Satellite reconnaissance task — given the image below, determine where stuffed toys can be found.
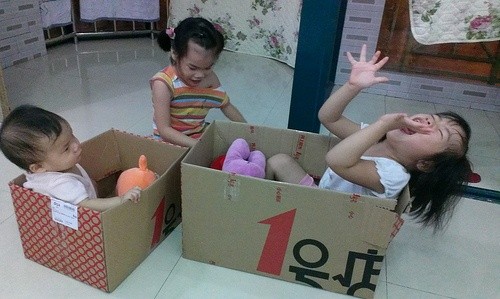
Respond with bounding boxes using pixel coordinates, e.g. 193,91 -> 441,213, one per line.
221,138 -> 266,179
116,155 -> 158,197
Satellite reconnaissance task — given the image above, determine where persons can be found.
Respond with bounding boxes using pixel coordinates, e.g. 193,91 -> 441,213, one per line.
266,43 -> 471,235
0,105 -> 143,212
148,17 -> 248,148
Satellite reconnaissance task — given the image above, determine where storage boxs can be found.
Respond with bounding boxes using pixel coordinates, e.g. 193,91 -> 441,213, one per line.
181,121 -> 411,299
9,128 -> 192,293
